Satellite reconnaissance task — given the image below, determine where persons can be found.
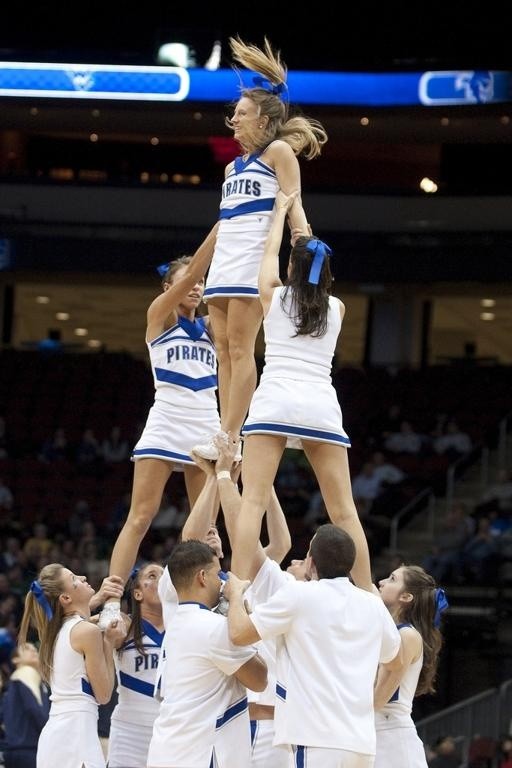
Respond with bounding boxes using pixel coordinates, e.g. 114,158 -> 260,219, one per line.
0,330 -> 512,768
216,188 -> 372,614
97,220 -> 221,637
193,33 -> 328,462
